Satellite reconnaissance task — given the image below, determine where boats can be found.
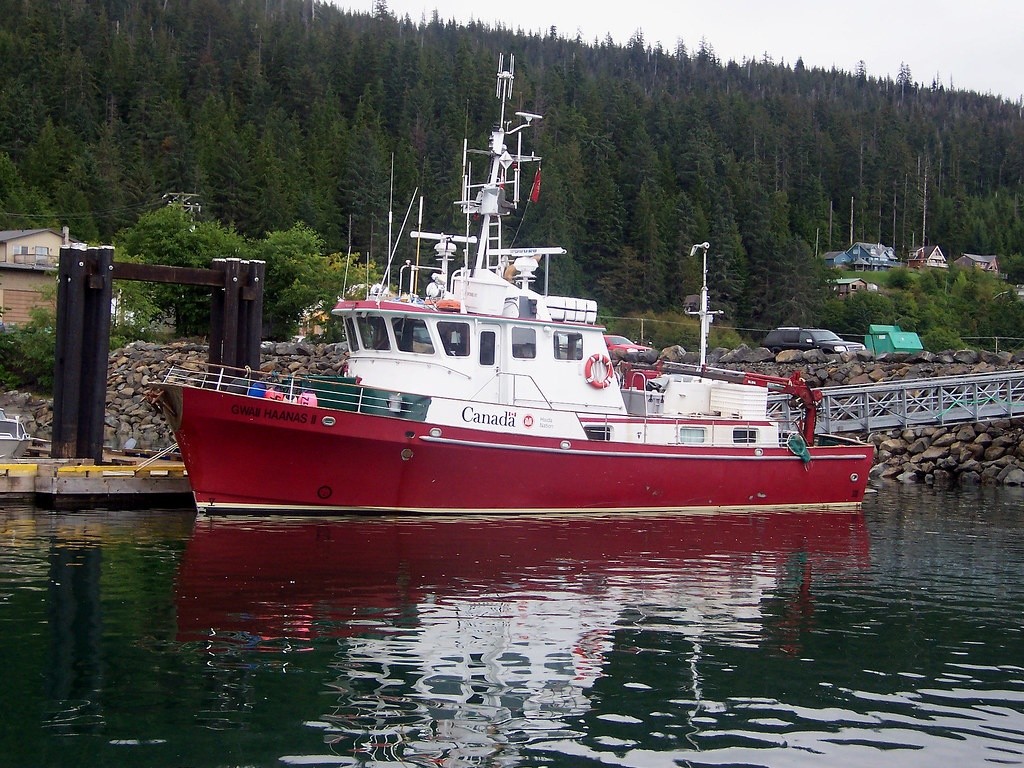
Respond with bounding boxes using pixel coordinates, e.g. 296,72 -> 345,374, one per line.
141,49 -> 876,515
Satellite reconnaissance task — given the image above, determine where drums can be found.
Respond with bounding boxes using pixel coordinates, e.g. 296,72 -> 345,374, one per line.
585,354 -> 613,388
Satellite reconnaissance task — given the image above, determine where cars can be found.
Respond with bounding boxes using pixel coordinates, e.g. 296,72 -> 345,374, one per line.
601,333 -> 653,356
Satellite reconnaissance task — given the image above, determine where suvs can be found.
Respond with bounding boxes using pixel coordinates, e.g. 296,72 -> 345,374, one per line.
759,327 -> 865,354
